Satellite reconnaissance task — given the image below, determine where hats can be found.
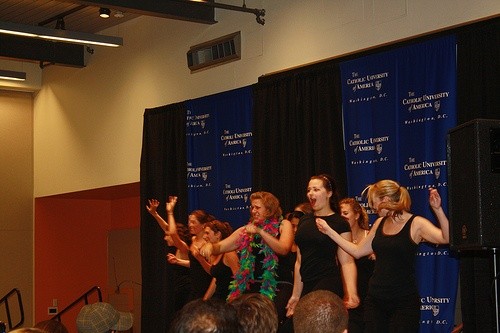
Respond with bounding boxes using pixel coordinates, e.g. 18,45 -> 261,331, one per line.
76,302 -> 134,333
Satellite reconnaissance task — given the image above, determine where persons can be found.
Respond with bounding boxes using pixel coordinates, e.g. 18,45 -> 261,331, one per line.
76,301 -> 135,333
204,221 -> 241,301
274,201 -> 312,333
314,187 -> 449,333
340,197 -> 376,333
229,292 -> 278,333
291,290 -> 348,333
167,297 -> 241,333
452,224 -> 475,245
285,174 -> 360,316
199,191 -> 293,304
166,210 -> 216,300
146,195 -> 193,324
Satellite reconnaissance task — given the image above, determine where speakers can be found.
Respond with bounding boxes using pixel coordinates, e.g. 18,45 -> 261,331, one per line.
446,118 -> 500,253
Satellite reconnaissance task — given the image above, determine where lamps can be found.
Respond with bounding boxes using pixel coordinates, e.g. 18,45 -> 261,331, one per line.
0,14 -> 123,49
99,8 -> 111,19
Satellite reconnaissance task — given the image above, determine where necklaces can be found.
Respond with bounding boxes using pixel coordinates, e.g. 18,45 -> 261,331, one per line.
351,230 -> 365,244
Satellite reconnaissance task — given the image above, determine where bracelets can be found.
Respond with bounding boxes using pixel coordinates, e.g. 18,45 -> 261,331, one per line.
167,212 -> 174,215
